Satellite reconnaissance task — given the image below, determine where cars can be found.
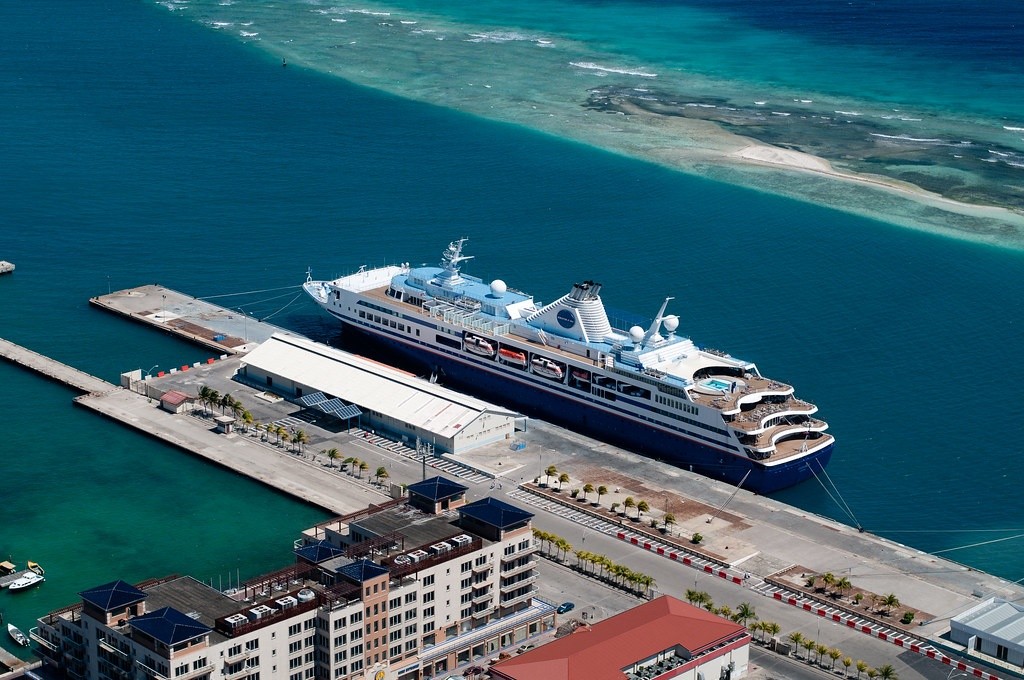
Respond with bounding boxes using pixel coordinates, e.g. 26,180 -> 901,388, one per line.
463,665 -> 484,678
517,643 -> 535,654
557,602 -> 575,614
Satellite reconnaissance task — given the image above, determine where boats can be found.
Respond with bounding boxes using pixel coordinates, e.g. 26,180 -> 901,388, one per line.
8,623 -> 31,647
8,571 -> 45,592
27,560 -> 44,576
302,233 -> 836,495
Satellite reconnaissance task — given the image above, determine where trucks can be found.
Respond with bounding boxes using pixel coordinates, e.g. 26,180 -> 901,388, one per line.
489,651 -> 512,664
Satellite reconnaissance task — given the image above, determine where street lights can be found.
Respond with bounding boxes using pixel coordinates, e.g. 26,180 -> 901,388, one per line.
303,419 -> 318,457
107,275 -> 110,297
238,307 -> 254,341
817,617 -> 820,643
219,388 -> 239,416
136,364 -> 161,403
